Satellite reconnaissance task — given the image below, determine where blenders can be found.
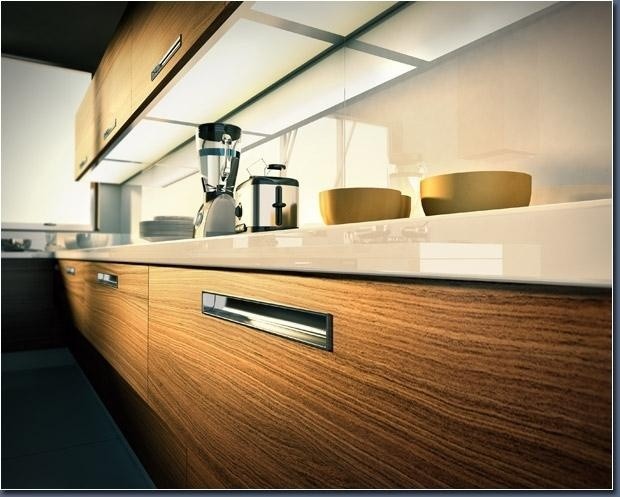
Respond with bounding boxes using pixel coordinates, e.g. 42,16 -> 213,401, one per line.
194,123 -> 242,239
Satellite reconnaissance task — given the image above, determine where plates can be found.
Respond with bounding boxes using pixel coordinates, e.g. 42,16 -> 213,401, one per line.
138,215 -> 194,242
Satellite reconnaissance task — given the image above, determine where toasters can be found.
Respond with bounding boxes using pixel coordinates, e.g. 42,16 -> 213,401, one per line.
234,164 -> 300,232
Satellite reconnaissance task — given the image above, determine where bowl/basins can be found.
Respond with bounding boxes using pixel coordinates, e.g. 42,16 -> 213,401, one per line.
319,188 -> 411,225
1,238 -> 32,251
420,171 -> 531,215
64,232 -> 111,248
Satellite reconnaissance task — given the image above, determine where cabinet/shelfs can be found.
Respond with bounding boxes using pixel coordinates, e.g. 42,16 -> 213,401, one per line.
74,0 -> 408,186
3,260 -> 63,353
55,260 -> 613,489
